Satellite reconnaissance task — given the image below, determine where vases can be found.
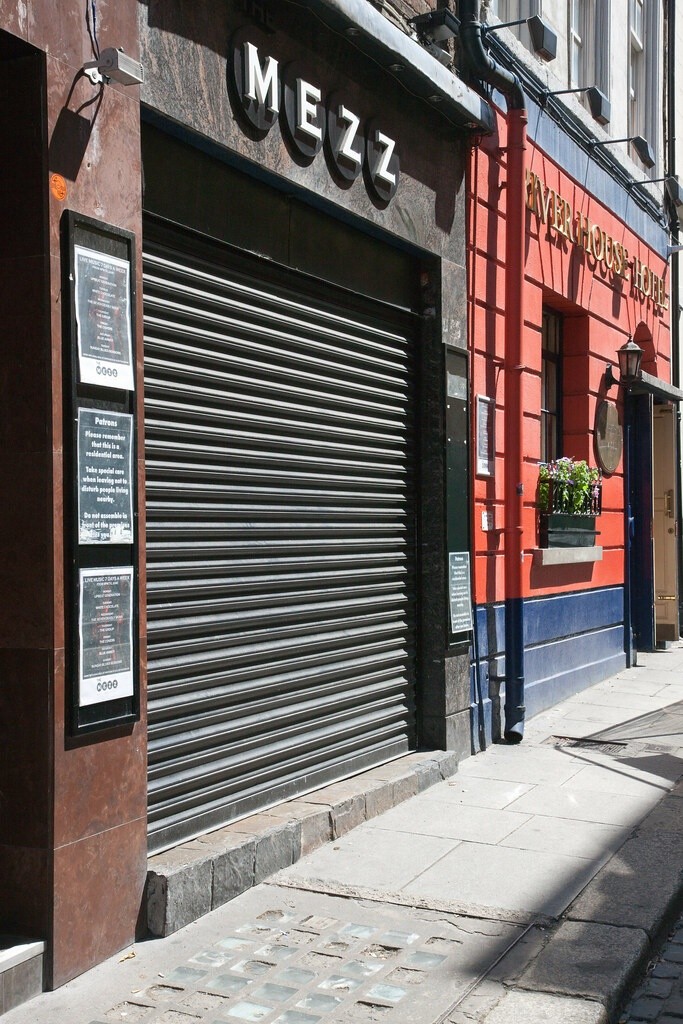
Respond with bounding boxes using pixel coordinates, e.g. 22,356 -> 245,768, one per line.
540,514 -> 596,548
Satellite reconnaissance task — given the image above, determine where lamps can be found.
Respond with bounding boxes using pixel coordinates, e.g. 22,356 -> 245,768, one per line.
629,176 -> 683,208
606,332 -> 645,390
482,14 -> 558,61
540,85 -> 611,125
589,135 -> 656,169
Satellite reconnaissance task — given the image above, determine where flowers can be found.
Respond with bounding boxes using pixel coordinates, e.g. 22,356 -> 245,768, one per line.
540,455 -> 603,517
